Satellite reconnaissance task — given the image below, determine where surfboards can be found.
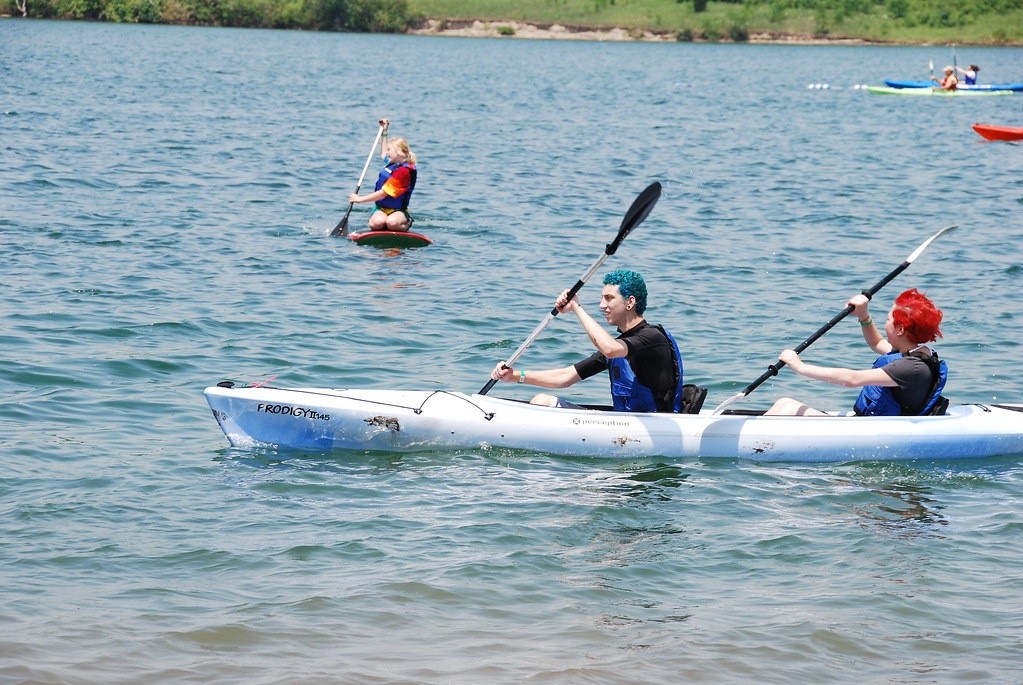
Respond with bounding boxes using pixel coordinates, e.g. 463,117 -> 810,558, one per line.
345,224 -> 433,247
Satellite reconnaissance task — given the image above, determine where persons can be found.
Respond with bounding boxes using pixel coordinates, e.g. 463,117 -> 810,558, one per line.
930,68 -> 958,92
491,270 -> 676,412
777,286 -> 944,416
349,119 -> 417,232
957,64 -> 980,85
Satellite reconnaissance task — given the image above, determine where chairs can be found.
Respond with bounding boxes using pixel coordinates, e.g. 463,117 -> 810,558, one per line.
681,383 -> 708,413
926,395 -> 950,416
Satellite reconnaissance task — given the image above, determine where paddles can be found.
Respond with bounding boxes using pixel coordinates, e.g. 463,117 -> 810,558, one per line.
929,59 -> 935,87
713,225 -> 961,416
329,126 -> 383,237
954,55 -> 957,77
478,180 -> 662,396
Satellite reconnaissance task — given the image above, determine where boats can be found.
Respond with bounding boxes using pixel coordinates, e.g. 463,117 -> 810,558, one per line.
203,384 -> 1022,464
973,124 -> 1023,142
867,81 -> 1023,96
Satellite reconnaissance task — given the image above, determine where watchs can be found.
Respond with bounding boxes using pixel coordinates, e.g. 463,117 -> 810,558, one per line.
517,369 -> 526,384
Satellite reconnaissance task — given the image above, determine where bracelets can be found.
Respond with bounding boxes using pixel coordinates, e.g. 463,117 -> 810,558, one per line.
858,314 -> 873,326
381,134 -> 387,136
